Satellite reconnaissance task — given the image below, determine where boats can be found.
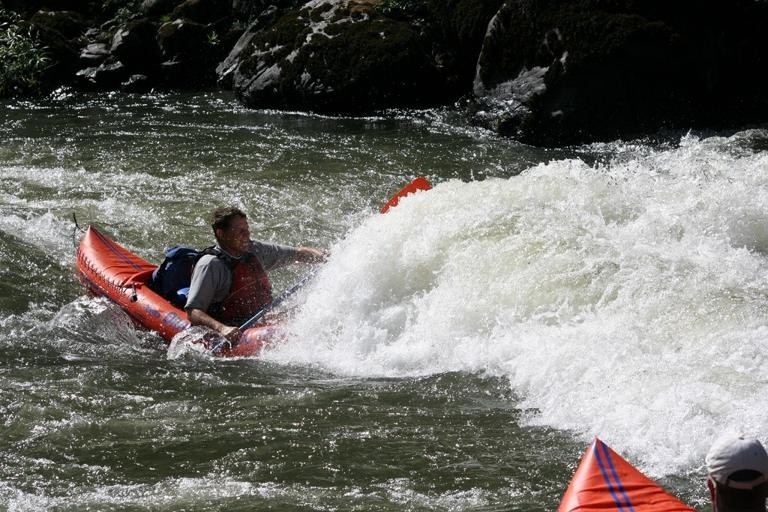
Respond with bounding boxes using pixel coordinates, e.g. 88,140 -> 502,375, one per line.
549,434 -> 696,512
73,218 -> 287,364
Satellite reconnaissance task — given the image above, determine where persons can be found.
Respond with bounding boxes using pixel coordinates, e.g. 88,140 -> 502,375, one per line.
184,203 -> 328,345
703,433 -> 768,512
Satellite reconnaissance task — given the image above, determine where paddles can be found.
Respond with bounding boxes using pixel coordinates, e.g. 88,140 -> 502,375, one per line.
210,177 -> 432,352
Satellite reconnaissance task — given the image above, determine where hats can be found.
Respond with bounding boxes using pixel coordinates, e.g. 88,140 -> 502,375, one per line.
705,433 -> 768,489
